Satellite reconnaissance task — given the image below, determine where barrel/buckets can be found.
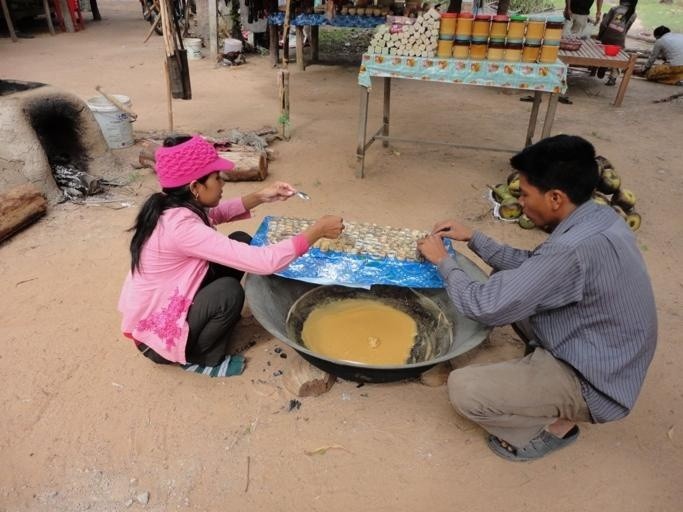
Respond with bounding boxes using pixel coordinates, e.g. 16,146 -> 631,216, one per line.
86,94 -> 135,150
223,38 -> 242,64
183,38 -> 202,61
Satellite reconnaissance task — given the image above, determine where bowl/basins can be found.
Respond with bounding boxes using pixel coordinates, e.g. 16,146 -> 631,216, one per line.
604,44 -> 620,56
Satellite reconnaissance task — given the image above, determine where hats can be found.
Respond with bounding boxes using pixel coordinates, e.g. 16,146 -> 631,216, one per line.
155,133 -> 234,189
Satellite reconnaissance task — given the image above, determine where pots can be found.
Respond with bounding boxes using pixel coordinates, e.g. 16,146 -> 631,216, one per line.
243,245 -> 499,387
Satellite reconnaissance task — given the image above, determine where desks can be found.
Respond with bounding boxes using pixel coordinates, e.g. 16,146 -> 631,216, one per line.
267,12 -> 386,72
352,53 -> 567,183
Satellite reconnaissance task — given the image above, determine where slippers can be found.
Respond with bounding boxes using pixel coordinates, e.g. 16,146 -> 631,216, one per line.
488,424 -> 580,462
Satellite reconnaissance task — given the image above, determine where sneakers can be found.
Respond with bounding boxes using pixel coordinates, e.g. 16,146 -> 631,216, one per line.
520,96 -> 541,101
597,67 -> 609,78
559,96 -> 573,105
608,78 -> 615,85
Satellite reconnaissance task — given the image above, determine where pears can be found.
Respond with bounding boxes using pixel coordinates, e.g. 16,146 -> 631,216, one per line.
486,156 -> 641,232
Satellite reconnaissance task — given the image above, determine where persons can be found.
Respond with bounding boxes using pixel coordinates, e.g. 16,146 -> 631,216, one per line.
596,0 -> 638,86
563,0 -> 603,34
415,134 -> 659,463
633,26 -> 683,87
116,133 -> 347,378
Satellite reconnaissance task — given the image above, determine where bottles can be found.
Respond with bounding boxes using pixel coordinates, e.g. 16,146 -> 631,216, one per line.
438,13 -> 564,65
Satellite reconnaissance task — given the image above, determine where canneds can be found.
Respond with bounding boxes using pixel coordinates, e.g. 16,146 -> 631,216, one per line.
438,11 -> 564,64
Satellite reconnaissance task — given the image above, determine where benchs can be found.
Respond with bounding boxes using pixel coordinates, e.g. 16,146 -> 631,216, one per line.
555,35 -> 638,108
